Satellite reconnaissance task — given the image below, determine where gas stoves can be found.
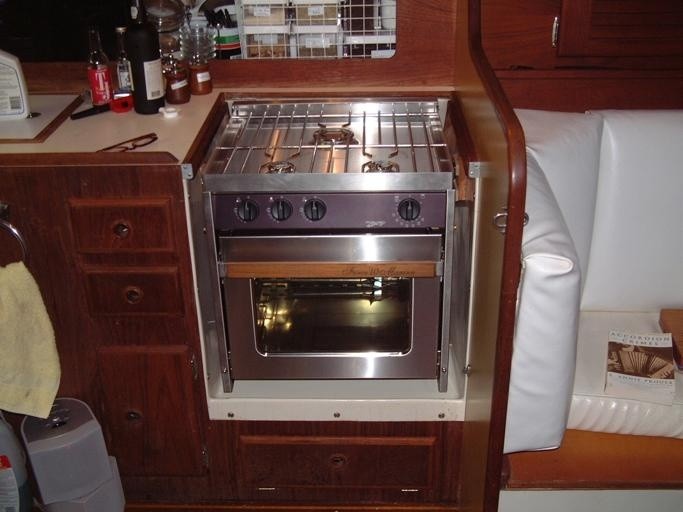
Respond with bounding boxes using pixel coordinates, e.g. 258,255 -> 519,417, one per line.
197,94 -> 457,231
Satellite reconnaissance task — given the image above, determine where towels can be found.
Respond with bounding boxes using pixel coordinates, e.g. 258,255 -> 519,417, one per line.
0,258 -> 63,424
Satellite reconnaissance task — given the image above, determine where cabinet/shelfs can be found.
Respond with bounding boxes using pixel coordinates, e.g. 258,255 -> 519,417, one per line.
228,423 -> 487,512
0,161 -> 226,512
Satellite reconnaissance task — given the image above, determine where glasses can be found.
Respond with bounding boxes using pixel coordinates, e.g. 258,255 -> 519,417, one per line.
96,133 -> 158,153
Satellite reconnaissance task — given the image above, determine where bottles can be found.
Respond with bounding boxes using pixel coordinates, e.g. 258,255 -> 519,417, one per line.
87,0 -> 210,114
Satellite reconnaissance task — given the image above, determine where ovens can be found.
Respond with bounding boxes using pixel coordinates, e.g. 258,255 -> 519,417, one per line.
217,234 -> 442,380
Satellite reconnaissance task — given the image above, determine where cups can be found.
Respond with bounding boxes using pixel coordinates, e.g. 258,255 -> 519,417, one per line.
217,19 -> 241,58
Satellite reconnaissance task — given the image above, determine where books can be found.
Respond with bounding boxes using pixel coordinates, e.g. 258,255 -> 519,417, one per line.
605,329 -> 675,406
